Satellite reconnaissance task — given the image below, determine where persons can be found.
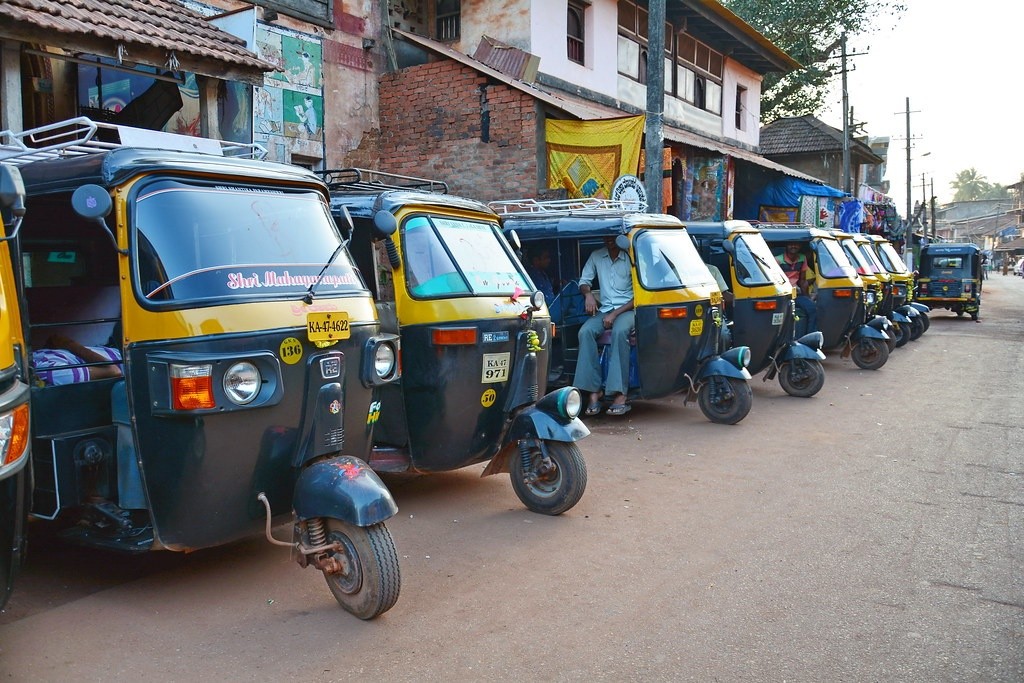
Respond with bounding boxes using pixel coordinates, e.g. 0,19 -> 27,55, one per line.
572,237 -> 647,416
27,334 -> 126,387
773,243 -> 827,335
525,245 -> 567,382
661,262 -> 732,353
980,254 -> 990,281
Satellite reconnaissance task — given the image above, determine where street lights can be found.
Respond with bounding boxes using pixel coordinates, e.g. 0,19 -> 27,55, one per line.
905,151 -> 932,247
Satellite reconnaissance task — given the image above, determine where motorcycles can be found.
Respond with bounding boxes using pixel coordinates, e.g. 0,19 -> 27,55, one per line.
749,220 -> 930,369
914,242 -> 985,319
312,168 -> 593,515
0,116 -> 402,621
486,197 -> 754,424
679,220 -> 828,398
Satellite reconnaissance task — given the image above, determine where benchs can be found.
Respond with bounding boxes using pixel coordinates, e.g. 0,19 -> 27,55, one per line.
24,284 -> 119,349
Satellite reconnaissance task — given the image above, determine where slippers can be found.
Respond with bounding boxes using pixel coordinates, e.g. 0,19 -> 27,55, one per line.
606,404 -> 631,414
585,403 -> 600,414
